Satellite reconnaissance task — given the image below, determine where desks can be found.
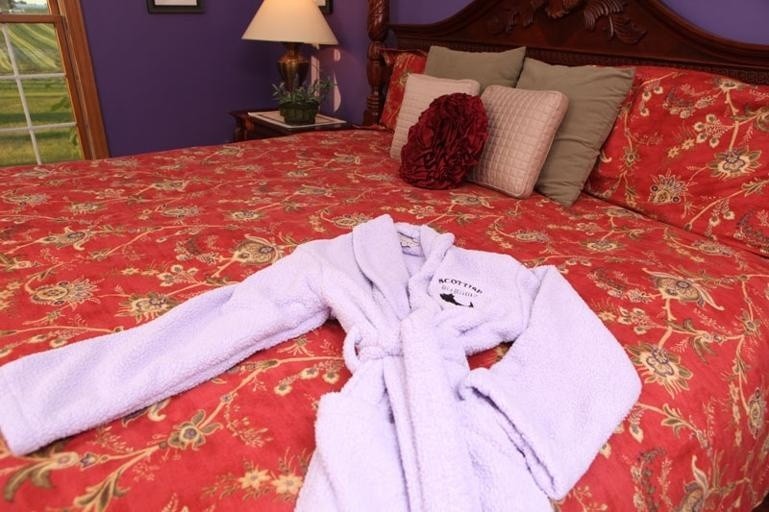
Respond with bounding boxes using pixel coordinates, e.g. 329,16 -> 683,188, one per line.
229,109 -> 349,142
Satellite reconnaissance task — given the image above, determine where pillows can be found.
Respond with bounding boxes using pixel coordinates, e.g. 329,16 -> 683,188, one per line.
421,42 -> 530,90
389,71 -> 481,175
579,61 -> 769,260
400,92 -> 492,194
459,87 -> 575,201
507,56 -> 640,207
379,50 -> 426,133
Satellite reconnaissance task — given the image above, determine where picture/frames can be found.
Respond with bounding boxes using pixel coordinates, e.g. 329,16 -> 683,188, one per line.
147,0 -> 204,14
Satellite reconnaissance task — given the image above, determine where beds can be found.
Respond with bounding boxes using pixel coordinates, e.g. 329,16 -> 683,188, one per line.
1,0 -> 769,512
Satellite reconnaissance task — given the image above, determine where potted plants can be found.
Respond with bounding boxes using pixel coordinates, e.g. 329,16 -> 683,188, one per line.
268,78 -> 336,124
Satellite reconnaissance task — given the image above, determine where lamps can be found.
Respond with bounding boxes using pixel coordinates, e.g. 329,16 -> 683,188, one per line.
236,0 -> 340,110
